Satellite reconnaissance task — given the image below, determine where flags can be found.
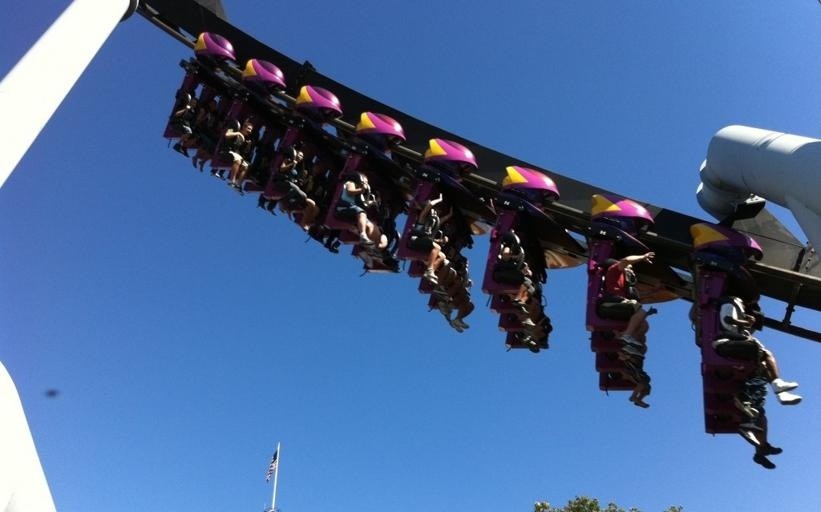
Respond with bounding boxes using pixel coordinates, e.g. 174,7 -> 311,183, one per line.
265,447 -> 278,485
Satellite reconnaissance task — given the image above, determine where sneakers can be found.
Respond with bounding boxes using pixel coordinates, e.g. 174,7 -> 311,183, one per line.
173,143 -> 241,190
772,378 -> 798,394
635,398 -> 650,408
256,204 -> 338,254
752,454 -> 776,469
777,392 -> 802,405
619,335 -> 650,385
738,429 -> 760,446
359,235 -> 401,274
423,269 -> 468,333
629,394 -> 636,401
755,444 -> 782,456
498,247 -> 539,353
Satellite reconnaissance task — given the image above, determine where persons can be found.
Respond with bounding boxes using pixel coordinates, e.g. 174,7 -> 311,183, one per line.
712,282 -> 801,469
171,90 -> 553,354
598,252 -> 657,409
